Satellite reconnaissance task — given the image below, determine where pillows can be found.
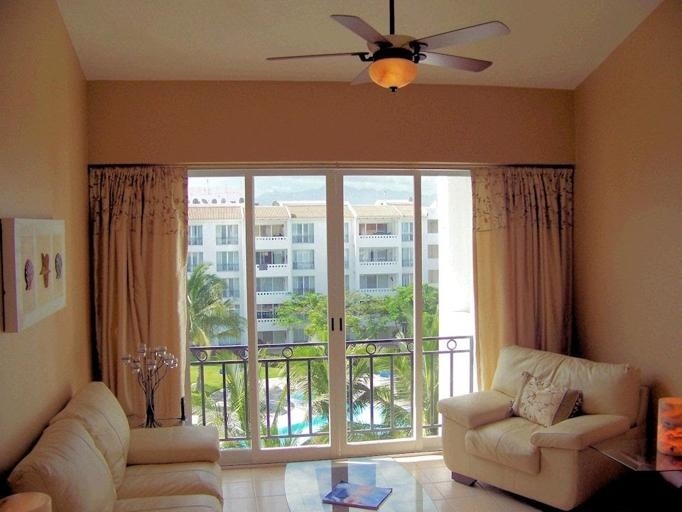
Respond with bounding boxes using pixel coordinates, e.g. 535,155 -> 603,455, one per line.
512,369 -> 583,427
9,418 -> 116,511
49,383 -> 130,491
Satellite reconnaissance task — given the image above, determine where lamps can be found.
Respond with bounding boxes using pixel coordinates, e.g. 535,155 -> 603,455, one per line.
657,396 -> 682,458
368,57 -> 418,92
121,342 -> 181,428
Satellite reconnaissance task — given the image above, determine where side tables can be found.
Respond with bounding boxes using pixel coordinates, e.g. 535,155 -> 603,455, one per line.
587,424 -> 682,511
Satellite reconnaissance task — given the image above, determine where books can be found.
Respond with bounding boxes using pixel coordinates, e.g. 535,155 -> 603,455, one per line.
323,479 -> 392,510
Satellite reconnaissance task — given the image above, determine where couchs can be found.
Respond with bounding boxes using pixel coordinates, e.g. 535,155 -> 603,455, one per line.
437,344 -> 650,511
1,382 -> 225,510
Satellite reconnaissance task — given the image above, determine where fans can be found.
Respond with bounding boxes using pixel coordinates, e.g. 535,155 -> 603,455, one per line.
265,1 -> 511,95
